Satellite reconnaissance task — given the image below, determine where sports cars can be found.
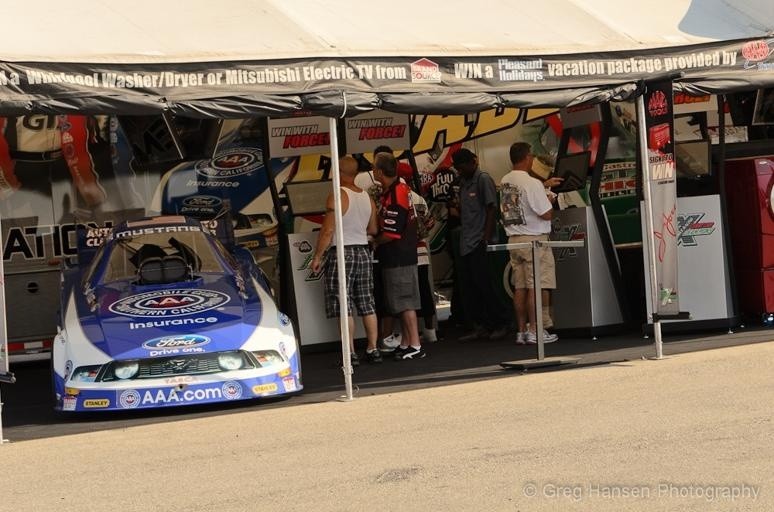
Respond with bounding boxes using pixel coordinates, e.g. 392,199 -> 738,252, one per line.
148,118 -> 297,250
51,214 -> 304,412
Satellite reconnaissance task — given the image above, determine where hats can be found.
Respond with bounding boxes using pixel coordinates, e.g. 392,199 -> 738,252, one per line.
452,148 -> 477,164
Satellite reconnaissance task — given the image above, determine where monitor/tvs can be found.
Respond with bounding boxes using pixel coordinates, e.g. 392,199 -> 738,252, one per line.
551,149 -> 591,194
674,139 -> 711,180
282,178 -> 332,217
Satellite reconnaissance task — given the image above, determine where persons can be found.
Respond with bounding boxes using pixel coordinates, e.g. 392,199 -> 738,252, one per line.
312,142 -> 566,366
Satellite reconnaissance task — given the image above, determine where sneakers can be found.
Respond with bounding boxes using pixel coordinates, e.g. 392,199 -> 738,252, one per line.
423,329 -> 437,342
516,328 -> 558,345
336,353 -> 359,366
365,347 -> 382,362
380,327 -> 426,361
